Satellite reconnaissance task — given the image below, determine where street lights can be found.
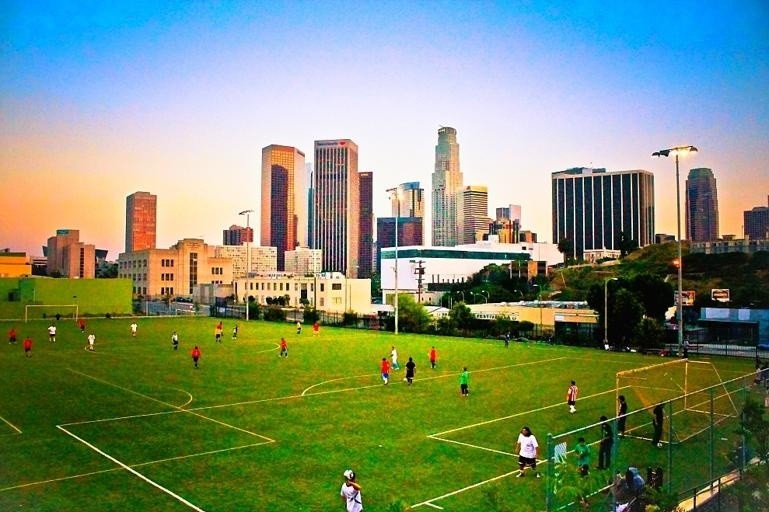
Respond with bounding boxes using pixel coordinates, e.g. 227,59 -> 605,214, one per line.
651,143 -> 704,361
422,280 -> 546,310
385,183 -> 410,336
344,265 -> 363,314
603,277 -> 620,353
237,208 -> 258,320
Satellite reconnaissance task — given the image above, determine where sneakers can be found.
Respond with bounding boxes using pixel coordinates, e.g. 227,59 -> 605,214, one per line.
568,409 -> 577,414
195,366 -> 199,369
596,466 -> 609,470
535,472 -> 541,478
89,349 -> 95,352
462,394 -> 468,397
216,335 -> 237,343
279,355 -> 288,358
384,367 -> 413,385
8,342 -> 17,345
26,354 -> 33,358
516,472 -> 525,478
431,365 -> 437,369
48,340 -> 55,343
174,347 -> 178,350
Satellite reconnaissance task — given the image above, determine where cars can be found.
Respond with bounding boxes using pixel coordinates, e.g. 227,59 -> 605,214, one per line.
757,340 -> 769,352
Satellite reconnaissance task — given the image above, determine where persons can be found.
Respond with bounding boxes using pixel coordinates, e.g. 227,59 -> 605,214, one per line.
171,331 -> 179,350
23,336 -> 32,358
296,320 -> 320,336
683,336 -> 692,358
514,382 -> 664,512
505,334 -> 510,347
130,322 -> 138,337
746,360 -> 769,393
79,319 -> 86,332
380,347 -> 416,386
9,327 -> 17,344
430,346 -> 436,369
88,332 -> 96,351
48,323 -> 56,342
279,337 -> 287,358
191,344 -> 201,368
340,470 -> 363,511
723,434 -> 749,471
214,321 -> 237,343
460,367 -> 469,397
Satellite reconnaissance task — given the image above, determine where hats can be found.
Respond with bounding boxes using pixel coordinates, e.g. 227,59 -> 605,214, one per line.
343,470 -> 355,481
